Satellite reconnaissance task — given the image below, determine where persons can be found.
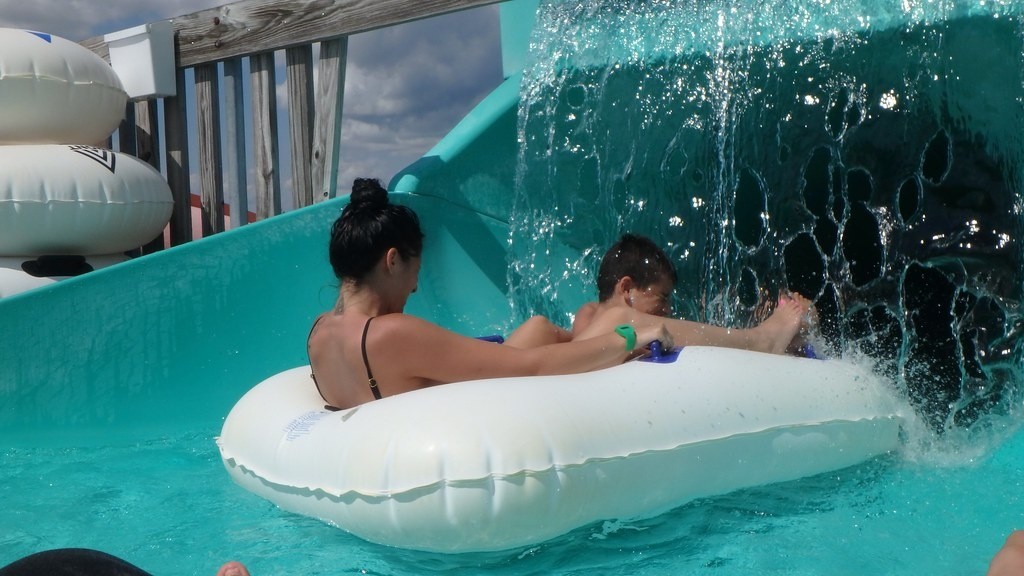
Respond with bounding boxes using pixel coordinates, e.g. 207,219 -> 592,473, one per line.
573,234 -> 819,354
308,176 -> 804,408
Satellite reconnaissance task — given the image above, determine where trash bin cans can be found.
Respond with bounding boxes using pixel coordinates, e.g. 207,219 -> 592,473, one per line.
104,21 -> 179,104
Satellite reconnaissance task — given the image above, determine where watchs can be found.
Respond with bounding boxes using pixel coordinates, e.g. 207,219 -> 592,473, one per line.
615,324 -> 636,350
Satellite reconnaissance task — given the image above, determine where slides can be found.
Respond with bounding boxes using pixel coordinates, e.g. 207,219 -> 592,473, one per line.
0,0 -> 1024,576
